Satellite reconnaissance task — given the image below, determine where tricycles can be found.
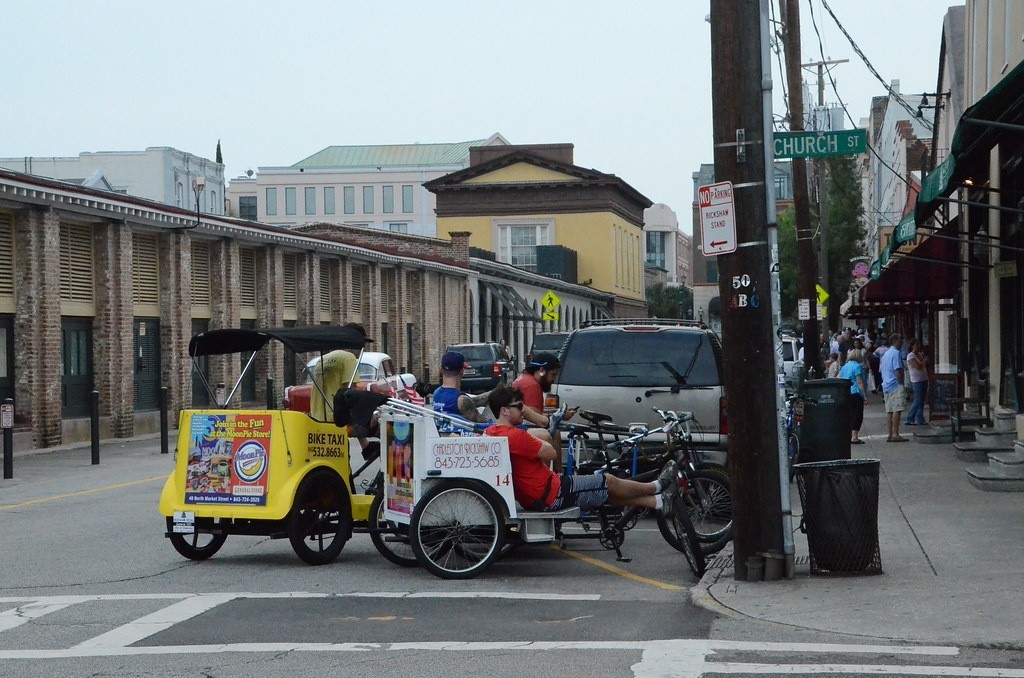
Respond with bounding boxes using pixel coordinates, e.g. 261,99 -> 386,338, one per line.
156,321 -> 737,580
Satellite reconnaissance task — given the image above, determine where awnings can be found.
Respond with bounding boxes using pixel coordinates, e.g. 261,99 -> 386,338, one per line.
868,57 -> 1024,279
480,281 -> 542,322
839,216 -> 962,319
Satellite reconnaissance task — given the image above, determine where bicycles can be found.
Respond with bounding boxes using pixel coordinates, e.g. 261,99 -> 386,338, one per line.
783,381 -> 819,483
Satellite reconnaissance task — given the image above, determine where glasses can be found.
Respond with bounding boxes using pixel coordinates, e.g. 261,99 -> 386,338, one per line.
509,403 -> 523,412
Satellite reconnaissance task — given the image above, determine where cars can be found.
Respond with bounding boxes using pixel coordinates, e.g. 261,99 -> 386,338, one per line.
282,350 -> 423,426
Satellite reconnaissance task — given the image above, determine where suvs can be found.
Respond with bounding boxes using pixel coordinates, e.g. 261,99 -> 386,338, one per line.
523,329 -> 572,371
437,340 -> 518,396
545,319 -> 725,513
781,330 -> 804,395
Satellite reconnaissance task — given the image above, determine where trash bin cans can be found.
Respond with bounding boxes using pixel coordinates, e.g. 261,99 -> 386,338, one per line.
792,457 -> 885,576
797,377 -> 864,463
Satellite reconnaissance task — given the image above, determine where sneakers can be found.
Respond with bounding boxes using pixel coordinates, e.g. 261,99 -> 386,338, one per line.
886,434 -> 910,442
657,460 -> 678,491
360,441 -> 381,461
544,403 -> 568,438
656,476 -> 681,520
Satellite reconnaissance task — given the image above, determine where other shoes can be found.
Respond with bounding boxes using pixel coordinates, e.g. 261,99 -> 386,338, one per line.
904,422 -> 928,425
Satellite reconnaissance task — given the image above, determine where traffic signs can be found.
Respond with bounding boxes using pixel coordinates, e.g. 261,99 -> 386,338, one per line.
697,181 -> 737,257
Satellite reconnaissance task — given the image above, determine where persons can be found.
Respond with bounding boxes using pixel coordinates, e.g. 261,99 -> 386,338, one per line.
797,326 -> 914,401
511,352 -> 576,472
500,339 -> 511,357
309,322 -> 381,461
432,351 -> 567,467
879,334 -> 909,442
903,339 -> 929,425
483,386 -> 681,517
836,349 -> 869,444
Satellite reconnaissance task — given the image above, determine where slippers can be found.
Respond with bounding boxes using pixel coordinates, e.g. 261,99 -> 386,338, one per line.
851,439 -> 865,444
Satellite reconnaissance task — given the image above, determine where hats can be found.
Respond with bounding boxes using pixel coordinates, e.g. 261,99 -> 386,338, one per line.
342,322 -> 375,343
